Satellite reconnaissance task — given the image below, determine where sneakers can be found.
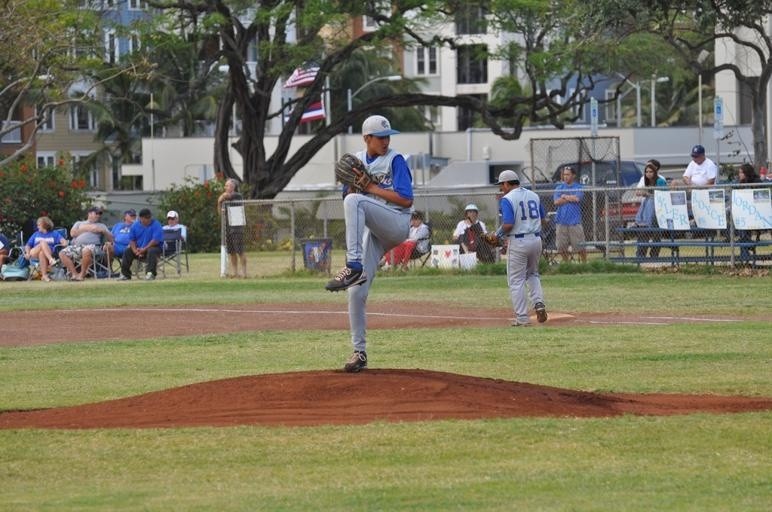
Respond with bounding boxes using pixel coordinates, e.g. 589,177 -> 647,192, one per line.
325,268 -> 367,293
145,271 -> 155,280
116,275 -> 131,281
512,318 -> 532,327
345,350 -> 367,373
536,303 -> 547,322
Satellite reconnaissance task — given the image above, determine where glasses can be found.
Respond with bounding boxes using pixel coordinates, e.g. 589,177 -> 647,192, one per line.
565,167 -> 571,170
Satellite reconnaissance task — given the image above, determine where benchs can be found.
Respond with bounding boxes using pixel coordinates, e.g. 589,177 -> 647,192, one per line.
577,227 -> 768,273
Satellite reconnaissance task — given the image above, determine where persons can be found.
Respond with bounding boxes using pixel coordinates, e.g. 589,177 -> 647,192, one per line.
379,210 -> 431,271
218,179 -> 247,279
486,170 -> 547,326
452,204 -> 496,264
0,206 -> 187,283
634,145 -> 765,257
326,115 -> 414,373
553,166 -> 588,264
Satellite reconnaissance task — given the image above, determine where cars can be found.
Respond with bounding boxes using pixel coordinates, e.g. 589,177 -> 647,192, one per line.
415,158 -> 606,222
667,179 -> 772,240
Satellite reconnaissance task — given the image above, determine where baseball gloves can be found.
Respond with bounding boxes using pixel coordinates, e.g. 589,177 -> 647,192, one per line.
481,231 -> 501,247
335,153 -> 371,191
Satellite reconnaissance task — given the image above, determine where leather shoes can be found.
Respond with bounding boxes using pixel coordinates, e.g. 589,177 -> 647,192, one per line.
41,259 -> 91,283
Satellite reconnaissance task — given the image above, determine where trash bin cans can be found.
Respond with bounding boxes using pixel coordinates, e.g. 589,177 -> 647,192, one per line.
301,239 -> 332,273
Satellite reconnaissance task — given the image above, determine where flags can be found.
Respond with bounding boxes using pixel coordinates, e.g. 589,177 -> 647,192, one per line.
284,59 -> 320,88
298,98 -> 326,124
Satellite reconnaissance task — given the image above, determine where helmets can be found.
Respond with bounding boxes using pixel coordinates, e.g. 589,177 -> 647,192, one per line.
464,203 -> 480,212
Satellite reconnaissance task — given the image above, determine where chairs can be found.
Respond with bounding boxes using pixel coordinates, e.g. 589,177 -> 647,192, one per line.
11,227 -> 190,279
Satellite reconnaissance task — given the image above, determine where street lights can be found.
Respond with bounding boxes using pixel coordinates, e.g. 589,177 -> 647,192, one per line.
347,73 -> 402,167
696,47 -> 709,125
638,74 -> 670,127
0,75 -> 53,97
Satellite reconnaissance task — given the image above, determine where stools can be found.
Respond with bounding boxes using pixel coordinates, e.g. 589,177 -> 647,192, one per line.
409,251 -> 430,269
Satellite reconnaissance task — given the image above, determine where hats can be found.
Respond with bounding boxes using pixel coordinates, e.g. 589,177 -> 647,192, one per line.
125,209 -> 136,217
361,114 -> 401,138
88,206 -> 103,214
167,210 -> 179,218
691,144 -> 705,156
494,170 -> 520,185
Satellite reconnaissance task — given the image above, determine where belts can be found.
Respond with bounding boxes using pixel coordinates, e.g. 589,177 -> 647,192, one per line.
515,232 -> 540,238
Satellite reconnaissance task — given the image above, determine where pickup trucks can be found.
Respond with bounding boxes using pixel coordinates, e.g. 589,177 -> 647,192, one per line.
522,159 -> 673,247
